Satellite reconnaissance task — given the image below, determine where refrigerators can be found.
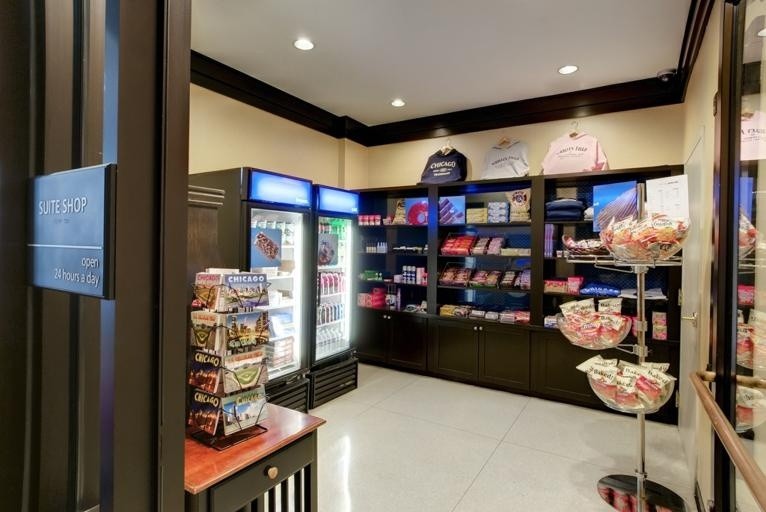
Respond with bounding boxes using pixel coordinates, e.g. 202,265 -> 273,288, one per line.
313,182 -> 360,409
190,166 -> 312,415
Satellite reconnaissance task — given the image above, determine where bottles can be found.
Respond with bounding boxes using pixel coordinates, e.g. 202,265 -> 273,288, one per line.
316,303 -> 345,324
317,269 -> 345,293
338,243 -> 346,265
319,218 -> 347,239
315,327 -> 349,355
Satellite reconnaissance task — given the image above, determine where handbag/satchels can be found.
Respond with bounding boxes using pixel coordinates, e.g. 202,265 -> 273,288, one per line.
392,200 -> 406,224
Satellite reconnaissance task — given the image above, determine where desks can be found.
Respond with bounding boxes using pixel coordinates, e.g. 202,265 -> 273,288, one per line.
185,398 -> 326,511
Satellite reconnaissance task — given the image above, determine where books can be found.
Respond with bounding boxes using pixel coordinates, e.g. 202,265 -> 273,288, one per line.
188,270 -> 269,435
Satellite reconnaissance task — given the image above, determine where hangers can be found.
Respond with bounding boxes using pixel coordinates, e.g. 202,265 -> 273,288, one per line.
495,133 -> 516,147
565,122 -> 581,137
438,138 -> 458,154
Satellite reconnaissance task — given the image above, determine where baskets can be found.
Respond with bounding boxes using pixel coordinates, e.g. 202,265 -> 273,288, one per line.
562,235 -> 609,255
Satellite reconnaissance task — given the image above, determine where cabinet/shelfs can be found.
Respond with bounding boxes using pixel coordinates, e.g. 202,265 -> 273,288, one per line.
433,176 -> 542,324
534,323 -> 679,426
352,183 -> 434,316
542,163 -> 686,328
426,318 -> 536,396
352,307 -> 428,375
